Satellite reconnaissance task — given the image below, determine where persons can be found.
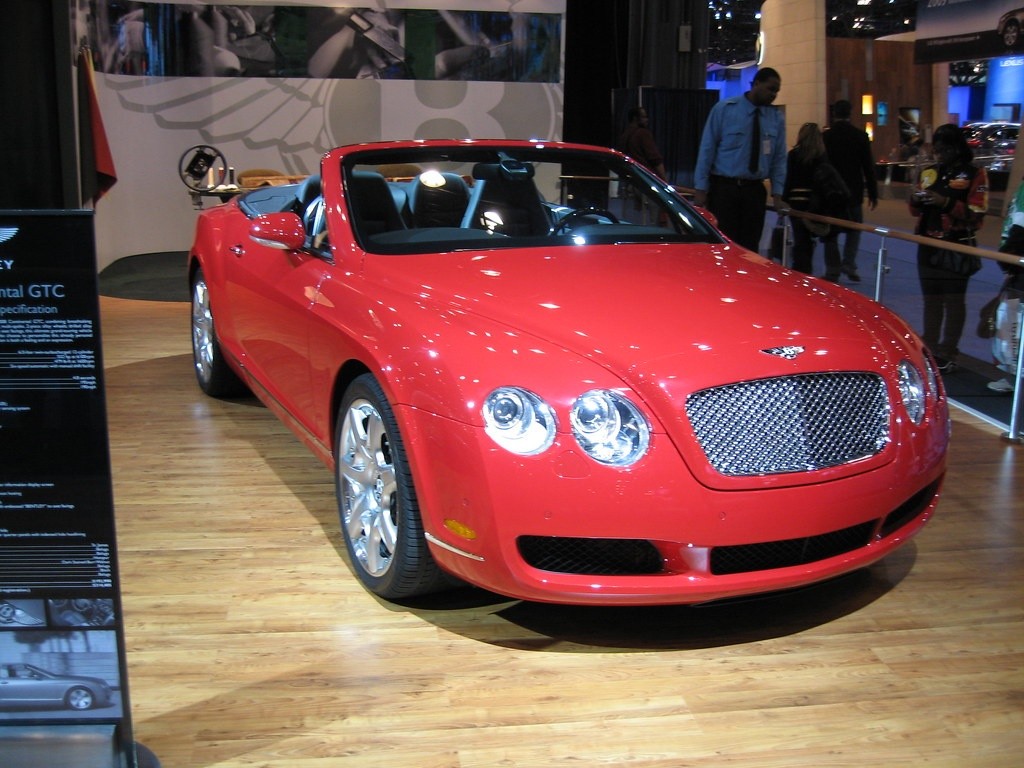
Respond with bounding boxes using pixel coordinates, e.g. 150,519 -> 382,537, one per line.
106,5 -> 528,80
622,68 -> 1024,372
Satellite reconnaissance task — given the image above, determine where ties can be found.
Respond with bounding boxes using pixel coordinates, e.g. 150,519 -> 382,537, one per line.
749,109 -> 760,173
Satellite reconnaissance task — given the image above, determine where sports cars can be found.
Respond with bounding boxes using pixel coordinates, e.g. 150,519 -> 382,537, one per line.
188,139 -> 953,621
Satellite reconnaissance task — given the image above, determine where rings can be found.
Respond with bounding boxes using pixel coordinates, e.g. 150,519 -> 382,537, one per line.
928,197 -> 932,201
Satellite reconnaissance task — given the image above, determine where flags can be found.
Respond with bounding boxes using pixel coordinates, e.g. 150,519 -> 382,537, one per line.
75,50 -> 119,210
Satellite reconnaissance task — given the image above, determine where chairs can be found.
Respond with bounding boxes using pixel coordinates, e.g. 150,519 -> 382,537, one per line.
410,173 -> 473,226
473,161 -> 552,241
295,168 -> 407,240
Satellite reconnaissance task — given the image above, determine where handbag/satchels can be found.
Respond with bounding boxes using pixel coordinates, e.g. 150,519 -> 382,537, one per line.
767,214 -> 786,259
941,230 -> 981,276
977,296 -> 1000,338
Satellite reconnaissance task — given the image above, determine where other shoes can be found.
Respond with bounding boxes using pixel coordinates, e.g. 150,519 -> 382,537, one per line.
933,355 -> 957,369
986,378 -> 1014,392
840,262 -> 861,283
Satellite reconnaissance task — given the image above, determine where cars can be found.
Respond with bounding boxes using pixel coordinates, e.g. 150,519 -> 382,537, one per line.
1,663 -> 114,710
961,120 -> 1022,176
